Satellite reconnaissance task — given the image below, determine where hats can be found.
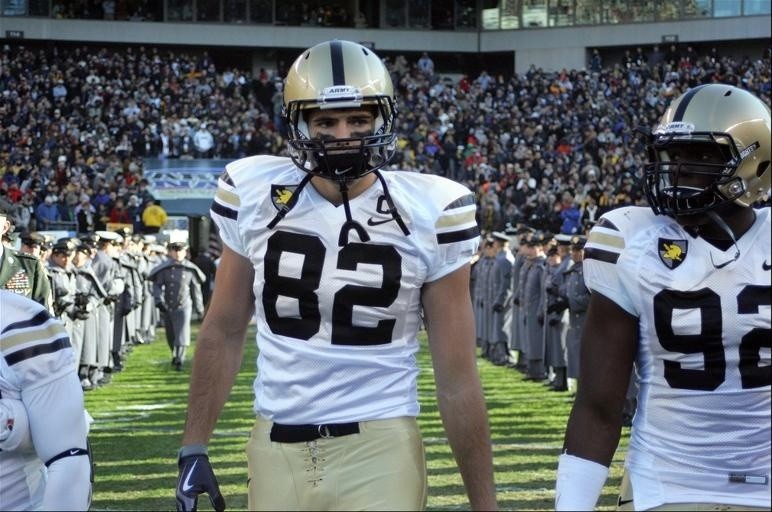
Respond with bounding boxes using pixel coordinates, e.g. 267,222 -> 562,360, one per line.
167,241 -> 191,250
52,241 -> 74,256
584,218 -> 599,229
518,226 -> 589,257
491,231 -> 511,243
18,231 -> 46,247
95,230 -> 120,243
57,233 -> 100,257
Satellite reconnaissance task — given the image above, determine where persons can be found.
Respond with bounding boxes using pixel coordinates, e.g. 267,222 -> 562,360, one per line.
176,39 -> 497,509
1,289 -> 95,511
556,83 -> 772,510
1,0 -> 771,425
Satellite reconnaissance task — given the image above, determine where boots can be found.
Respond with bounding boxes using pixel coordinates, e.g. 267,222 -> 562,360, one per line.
481,347 -> 570,392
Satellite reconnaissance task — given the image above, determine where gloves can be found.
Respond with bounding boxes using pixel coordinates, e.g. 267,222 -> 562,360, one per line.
197,310 -> 205,320
156,302 -> 172,314
514,298 -> 520,308
175,443 -> 226,512
537,283 -> 570,328
54,288 -> 142,321
493,304 -> 504,314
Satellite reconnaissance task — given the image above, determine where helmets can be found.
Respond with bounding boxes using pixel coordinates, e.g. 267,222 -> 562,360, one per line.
643,82 -> 771,221
283,39 -> 399,182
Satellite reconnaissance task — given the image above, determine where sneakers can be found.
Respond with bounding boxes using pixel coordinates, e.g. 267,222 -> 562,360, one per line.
81,335 -> 154,392
172,357 -> 178,366
176,361 -> 184,371
